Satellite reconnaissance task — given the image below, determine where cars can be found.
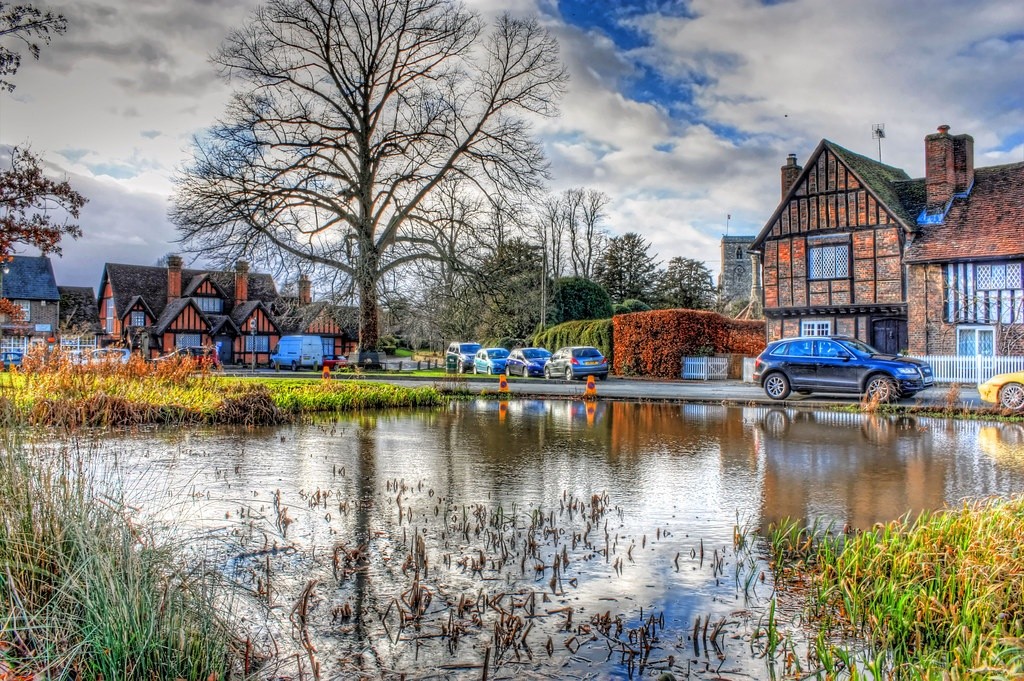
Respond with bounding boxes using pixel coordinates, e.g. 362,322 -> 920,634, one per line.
505,346 -> 553,378
0,352 -> 28,371
977,369 -> 1024,411
322,354 -> 348,371
543,346 -> 609,381
472,347 -> 511,375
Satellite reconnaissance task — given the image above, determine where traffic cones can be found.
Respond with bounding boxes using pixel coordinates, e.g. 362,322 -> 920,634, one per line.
497,372 -> 511,393
584,375 -> 596,395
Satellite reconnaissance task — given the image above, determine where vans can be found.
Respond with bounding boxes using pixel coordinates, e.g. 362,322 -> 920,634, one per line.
155,347 -> 220,372
76,347 -> 132,365
270,334 -> 323,369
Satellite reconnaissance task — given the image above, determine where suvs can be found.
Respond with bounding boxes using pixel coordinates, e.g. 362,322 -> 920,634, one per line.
446,342 -> 482,373
757,405 -> 921,447
753,335 -> 935,405
